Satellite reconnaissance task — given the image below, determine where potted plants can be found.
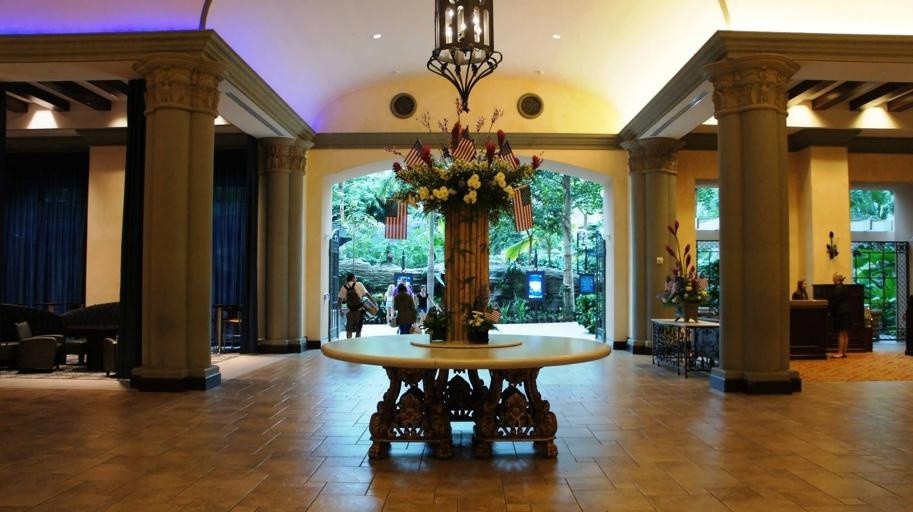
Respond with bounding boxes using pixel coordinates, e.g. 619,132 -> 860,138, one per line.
418,306 -> 456,343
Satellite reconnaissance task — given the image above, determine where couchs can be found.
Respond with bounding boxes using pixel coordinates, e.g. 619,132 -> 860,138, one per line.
0,302 -> 120,369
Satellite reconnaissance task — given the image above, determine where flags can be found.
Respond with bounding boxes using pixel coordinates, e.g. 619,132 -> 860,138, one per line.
500,141 -> 518,170
452,129 -> 475,162
513,184 -> 533,232
699,273 -> 707,287
664,276 -> 674,290
404,140 -> 432,178
483,306 -> 502,323
385,198 -> 407,240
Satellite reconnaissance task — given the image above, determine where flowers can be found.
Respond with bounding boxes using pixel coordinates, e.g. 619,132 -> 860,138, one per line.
656,219 -> 714,308
381,96 -> 545,228
463,296 -> 498,333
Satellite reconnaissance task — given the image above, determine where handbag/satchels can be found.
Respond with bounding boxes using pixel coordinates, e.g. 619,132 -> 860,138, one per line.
391,317 -> 397,327
409,327 -> 421,334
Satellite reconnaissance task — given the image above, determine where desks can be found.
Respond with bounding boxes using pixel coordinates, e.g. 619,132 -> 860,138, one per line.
650,318 -> 720,378
320,334 -> 611,461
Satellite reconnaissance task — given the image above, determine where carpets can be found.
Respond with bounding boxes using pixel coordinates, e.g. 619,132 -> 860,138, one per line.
0,353 -> 116,379
789,350 -> 912,383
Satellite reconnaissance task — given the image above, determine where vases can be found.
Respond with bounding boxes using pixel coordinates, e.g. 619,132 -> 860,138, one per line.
675,301 -> 698,323
467,328 -> 489,343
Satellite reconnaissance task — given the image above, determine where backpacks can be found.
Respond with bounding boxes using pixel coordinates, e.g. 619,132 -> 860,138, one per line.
344,282 -> 362,311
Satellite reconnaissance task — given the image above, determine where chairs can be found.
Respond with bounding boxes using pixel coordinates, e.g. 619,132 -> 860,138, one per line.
12,320 -> 65,372
102,334 -> 118,377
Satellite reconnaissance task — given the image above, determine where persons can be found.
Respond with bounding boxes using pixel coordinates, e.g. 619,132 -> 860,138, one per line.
829,271 -> 852,358
338,272 -> 437,339
793,278 -> 810,300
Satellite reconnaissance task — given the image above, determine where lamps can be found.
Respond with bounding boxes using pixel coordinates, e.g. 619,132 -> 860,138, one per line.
427,0 -> 503,114
